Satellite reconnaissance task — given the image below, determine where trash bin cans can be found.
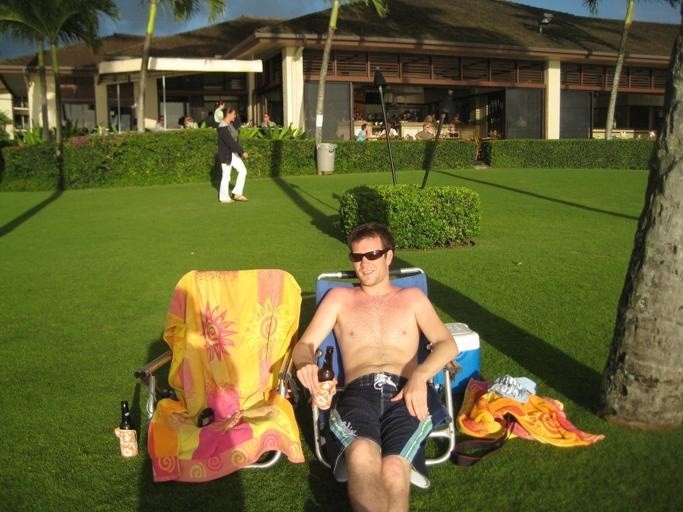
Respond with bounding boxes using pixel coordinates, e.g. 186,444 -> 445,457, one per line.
316,143 -> 337,175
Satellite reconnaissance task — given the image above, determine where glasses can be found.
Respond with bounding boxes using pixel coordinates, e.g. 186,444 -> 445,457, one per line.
346,247 -> 390,263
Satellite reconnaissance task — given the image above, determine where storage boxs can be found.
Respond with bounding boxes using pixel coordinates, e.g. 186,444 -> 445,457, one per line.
433,321 -> 481,391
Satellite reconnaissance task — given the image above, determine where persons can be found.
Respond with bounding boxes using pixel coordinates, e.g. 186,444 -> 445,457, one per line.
212,99 -> 224,128
182,114 -> 198,128
415,123 -> 435,140
288,221 -> 460,512
445,122 -> 459,137
233,111 -> 245,129
355,123 -> 371,141
450,113 -> 462,124
378,121 -> 399,140
155,115 -> 164,129
422,111 -> 440,124
259,113 -> 277,127
215,106 -> 249,204
354,111 -> 363,120
401,111 -> 418,122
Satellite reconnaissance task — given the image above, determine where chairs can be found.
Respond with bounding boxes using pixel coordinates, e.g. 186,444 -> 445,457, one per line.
134,269 -> 306,483
308,267 -> 457,470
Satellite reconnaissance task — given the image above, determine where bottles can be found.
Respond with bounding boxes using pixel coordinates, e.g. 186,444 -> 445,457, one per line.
118,400 -> 139,458
316,345 -> 335,414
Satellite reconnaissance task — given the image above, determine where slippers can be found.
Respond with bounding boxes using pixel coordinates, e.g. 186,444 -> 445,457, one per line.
234,195 -> 250,202
